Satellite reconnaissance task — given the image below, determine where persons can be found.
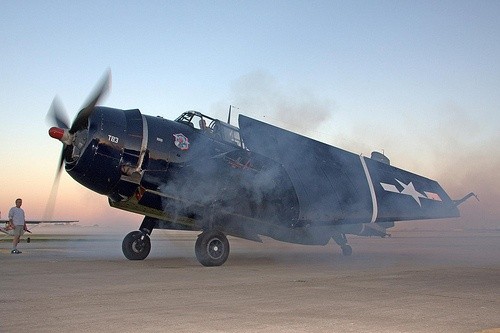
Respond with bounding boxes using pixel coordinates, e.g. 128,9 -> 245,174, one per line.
199,119 -> 214,139
8,198 -> 27,253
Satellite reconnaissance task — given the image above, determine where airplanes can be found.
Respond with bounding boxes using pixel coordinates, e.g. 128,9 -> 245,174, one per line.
43,66 -> 481,268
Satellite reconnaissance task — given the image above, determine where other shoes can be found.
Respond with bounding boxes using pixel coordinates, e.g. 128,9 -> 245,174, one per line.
11,250 -> 22,254
